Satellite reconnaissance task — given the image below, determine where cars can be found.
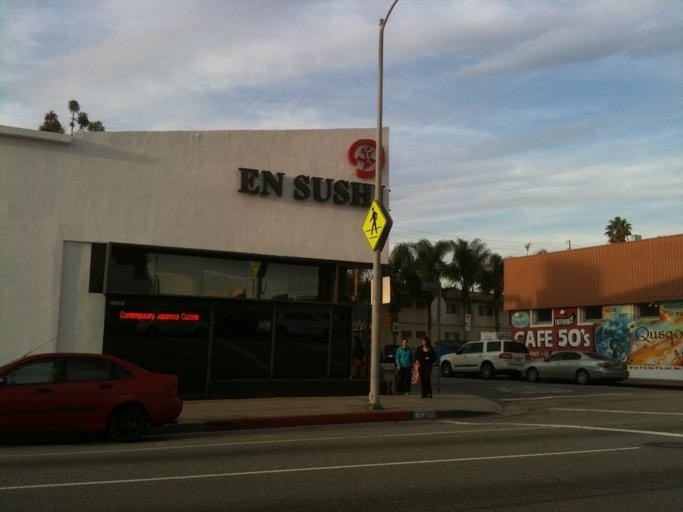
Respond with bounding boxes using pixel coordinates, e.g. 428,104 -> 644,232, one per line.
521,351 -> 629,385
0,352 -> 183,442
380,345 -> 401,364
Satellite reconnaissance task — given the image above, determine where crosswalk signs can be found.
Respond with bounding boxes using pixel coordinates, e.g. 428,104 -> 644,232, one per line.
361,200 -> 387,252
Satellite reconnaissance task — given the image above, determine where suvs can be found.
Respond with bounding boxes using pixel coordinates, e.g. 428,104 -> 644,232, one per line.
438,340 -> 532,378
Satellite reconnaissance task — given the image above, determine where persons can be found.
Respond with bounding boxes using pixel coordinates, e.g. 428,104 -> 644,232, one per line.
352,334 -> 362,362
414,335 -> 438,398
394,337 -> 413,396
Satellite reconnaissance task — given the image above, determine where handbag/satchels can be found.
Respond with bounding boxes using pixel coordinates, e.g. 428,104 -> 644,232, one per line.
411,364 -> 420,385
